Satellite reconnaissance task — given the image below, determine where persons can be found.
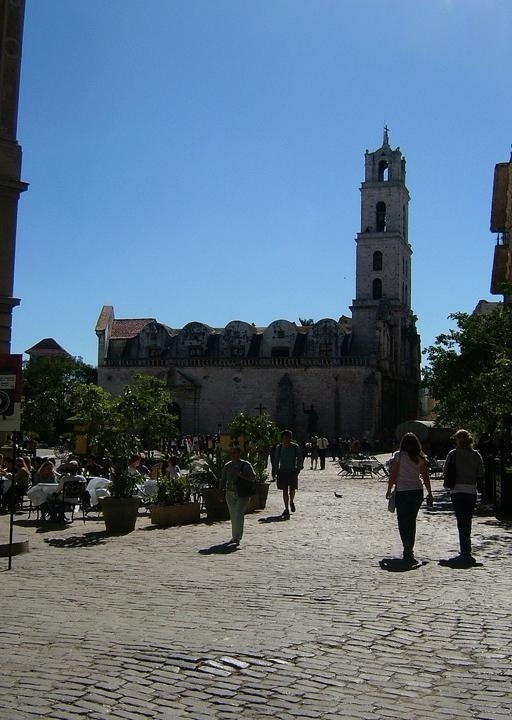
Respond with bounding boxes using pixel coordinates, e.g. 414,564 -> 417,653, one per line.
441,429 -> 485,557
248,435 -> 366,483
0,432 -> 221,519
274,430 -> 304,515
219,446 -> 257,547
385,432 -> 434,566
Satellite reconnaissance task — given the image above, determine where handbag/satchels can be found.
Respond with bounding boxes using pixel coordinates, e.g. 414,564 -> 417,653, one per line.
388,491 -> 396,513
444,449 -> 457,488
237,479 -> 257,497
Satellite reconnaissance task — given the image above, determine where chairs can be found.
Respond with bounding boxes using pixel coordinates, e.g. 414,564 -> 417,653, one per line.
0,464 -> 195,528
332,449 -> 443,478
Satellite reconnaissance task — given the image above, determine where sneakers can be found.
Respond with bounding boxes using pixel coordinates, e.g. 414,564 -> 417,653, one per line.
284,509 -> 289,519
290,503 -> 295,512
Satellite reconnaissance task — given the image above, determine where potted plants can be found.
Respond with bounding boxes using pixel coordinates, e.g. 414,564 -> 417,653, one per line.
97,406 -> 280,535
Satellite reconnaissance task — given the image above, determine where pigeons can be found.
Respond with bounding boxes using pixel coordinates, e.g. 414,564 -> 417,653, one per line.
332,491 -> 343,499
